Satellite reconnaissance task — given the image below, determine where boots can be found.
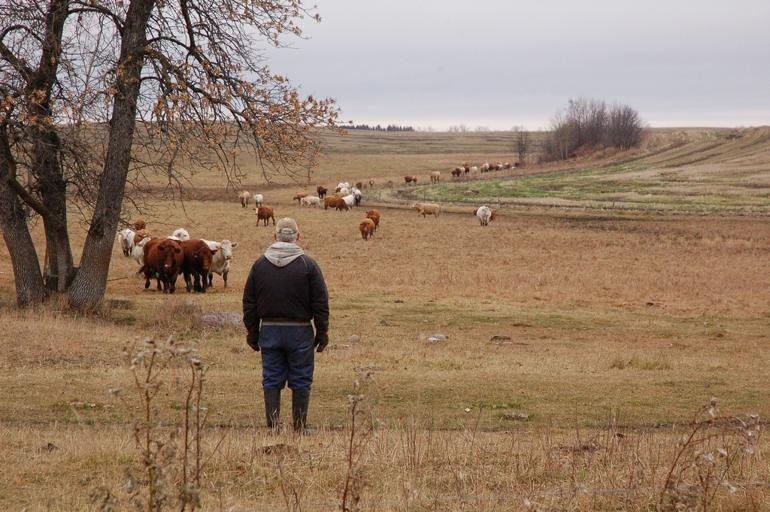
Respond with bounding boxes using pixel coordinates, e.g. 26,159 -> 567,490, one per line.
264,389 -> 281,436
292,390 -> 312,436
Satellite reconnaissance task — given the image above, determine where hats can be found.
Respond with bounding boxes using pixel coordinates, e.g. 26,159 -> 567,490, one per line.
276,217 -> 298,235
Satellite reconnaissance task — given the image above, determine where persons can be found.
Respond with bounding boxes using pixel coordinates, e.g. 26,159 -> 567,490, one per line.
243,217 -> 330,431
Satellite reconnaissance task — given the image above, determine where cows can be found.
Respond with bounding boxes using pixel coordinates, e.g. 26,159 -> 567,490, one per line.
413,203 -> 441,218
450,161 -> 520,177
238,191 -> 250,208
117,219 -> 237,293
252,206 -> 275,227
253,194 -> 263,208
430,171 -> 440,184
404,176 -> 418,186
359,209 -> 380,240
472,205 -> 497,227
293,182 -> 363,212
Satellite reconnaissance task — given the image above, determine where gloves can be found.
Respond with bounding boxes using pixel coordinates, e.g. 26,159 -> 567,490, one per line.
314,329 -> 329,352
245,328 -> 261,353
